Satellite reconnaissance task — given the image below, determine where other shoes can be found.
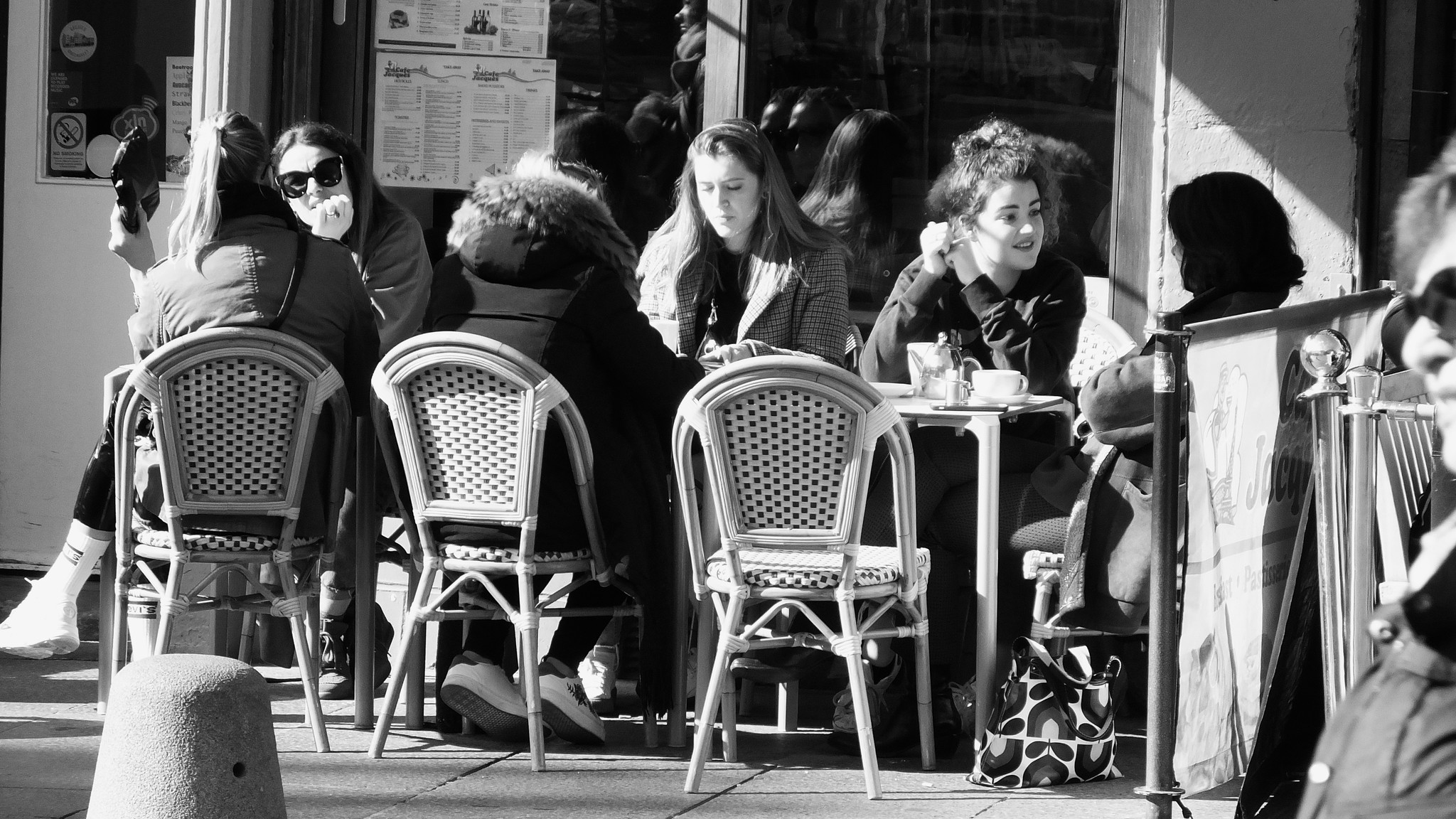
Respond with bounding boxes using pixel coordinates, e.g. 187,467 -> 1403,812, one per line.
633,645 -> 696,699
948,646 -> 1013,734
729,646 -> 832,683
832,650 -> 902,734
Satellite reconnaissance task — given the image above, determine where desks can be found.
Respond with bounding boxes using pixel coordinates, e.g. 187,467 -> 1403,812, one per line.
884,392 -> 1063,771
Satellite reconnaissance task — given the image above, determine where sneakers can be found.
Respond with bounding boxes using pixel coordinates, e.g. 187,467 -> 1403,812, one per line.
1,577 -> 79,659
577,649 -> 618,713
440,656 -> 554,743
524,661 -> 606,746
316,617 -> 352,699
376,622 -> 394,689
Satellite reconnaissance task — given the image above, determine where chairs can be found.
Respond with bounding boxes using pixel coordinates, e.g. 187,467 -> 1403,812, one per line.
369,330 -> 659,770
114,326 -> 352,753
669,356 -> 934,799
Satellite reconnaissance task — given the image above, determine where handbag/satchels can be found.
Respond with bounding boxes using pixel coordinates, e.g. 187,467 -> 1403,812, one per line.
110,125 -> 160,236
967,636 -> 1123,790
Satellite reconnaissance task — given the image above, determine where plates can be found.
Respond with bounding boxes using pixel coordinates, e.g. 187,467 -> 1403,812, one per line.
968,392 -> 1033,406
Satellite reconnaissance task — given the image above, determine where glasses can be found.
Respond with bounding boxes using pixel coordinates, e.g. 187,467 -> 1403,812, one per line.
1381,264 -> 1455,372
273,154 -> 344,198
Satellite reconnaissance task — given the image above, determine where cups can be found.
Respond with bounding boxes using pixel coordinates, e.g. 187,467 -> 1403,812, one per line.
649,319 -> 679,355
971,369 -> 1030,396
945,381 -> 975,406
906,342 -> 937,397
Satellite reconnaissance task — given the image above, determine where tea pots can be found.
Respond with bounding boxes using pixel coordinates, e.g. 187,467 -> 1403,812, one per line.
909,332 -> 983,401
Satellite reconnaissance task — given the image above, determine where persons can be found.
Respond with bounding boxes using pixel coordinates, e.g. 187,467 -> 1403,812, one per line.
633,0 -> 904,323
581,119 -> 857,709
269,120 -> 440,700
0,111 -> 364,665
831,114 -> 1089,738
430,110 -> 708,745
1289,131 -> 1456,819
949,169 -> 1306,768
